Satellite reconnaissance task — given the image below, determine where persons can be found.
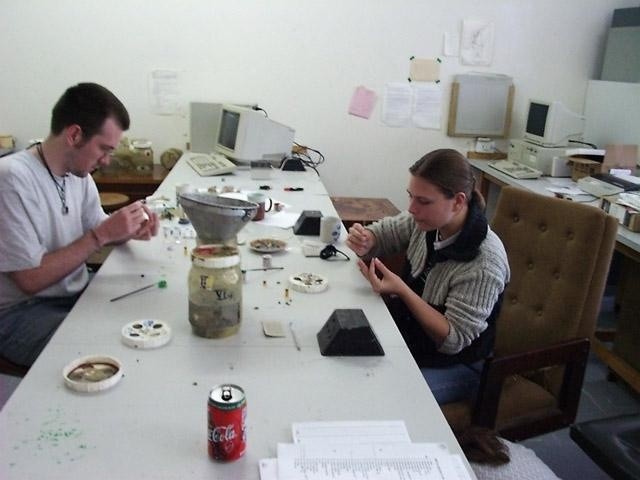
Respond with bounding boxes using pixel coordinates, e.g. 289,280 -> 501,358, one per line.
1,79 -> 160,371
345,147 -> 513,371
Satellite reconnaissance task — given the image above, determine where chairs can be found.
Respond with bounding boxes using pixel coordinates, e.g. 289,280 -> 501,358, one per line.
446,187 -> 620,444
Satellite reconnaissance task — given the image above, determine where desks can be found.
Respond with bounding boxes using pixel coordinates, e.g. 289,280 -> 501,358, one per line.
0,141 -> 486,480
465,152 -> 639,393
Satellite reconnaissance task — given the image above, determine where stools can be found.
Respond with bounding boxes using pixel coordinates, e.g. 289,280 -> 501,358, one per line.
332,194 -> 399,223
96,191 -> 129,215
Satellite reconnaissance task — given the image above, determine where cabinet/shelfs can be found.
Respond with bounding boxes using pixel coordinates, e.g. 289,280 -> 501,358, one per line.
90,164 -> 172,216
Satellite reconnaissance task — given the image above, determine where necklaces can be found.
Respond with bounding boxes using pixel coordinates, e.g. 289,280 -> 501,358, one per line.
36,141 -> 72,218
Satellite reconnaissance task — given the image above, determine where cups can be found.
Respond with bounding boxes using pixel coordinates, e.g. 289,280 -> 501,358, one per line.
176,183 -> 190,207
247,192 -> 272,221
319,216 -> 341,243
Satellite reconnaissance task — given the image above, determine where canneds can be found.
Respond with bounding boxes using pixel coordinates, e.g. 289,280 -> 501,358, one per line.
207,384 -> 246,463
129,139 -> 153,176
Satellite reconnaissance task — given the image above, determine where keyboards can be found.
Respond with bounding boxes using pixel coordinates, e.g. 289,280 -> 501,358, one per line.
186,151 -> 236,177
487,158 -> 543,181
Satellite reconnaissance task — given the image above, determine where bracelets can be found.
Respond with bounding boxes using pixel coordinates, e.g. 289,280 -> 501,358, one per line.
89,229 -> 104,255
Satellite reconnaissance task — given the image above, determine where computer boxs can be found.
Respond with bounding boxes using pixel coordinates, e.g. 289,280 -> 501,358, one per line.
190,101 -> 223,154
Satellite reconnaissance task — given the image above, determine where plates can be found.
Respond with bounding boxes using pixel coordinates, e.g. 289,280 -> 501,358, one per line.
63,354 -> 125,394
248,237 -> 287,253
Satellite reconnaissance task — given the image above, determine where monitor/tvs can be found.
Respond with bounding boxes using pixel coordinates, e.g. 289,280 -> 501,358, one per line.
216,106 -> 295,170
524,98 -> 585,148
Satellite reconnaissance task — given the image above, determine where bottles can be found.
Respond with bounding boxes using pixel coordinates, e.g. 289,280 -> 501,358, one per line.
132,141 -> 153,176
